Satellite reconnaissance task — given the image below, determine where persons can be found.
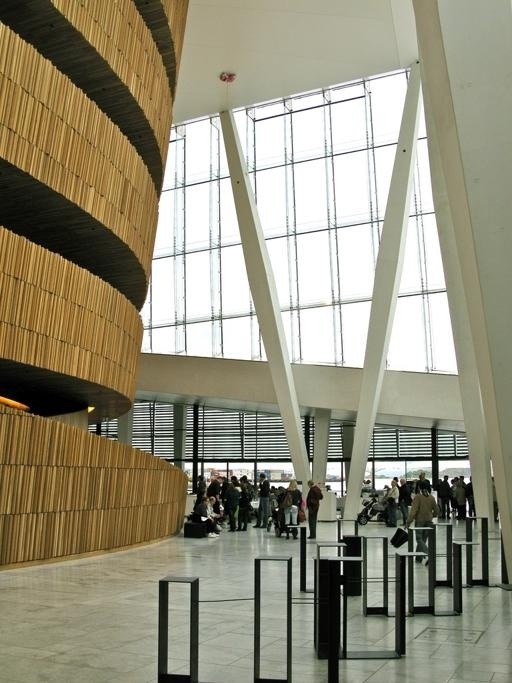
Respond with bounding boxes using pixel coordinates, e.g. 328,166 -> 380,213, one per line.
386,474 -> 498,527
192,473 -> 323,539
405,482 -> 440,566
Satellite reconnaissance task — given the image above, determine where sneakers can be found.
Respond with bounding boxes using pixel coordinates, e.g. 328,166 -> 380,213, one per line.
208,524 -> 221,537
228,529 -> 247,531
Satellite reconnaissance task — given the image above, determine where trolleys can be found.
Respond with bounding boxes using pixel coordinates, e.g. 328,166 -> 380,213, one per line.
272,507 -> 298,537
357,498 -> 378,525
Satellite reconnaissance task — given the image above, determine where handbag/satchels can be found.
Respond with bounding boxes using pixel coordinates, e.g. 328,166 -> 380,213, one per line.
391,528 -> 409,548
285,495 -> 293,506
451,497 -> 458,507
300,511 -> 305,522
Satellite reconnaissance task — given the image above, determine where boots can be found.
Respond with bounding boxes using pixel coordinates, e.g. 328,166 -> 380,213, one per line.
260,523 -> 268,528
253,522 -> 261,527
